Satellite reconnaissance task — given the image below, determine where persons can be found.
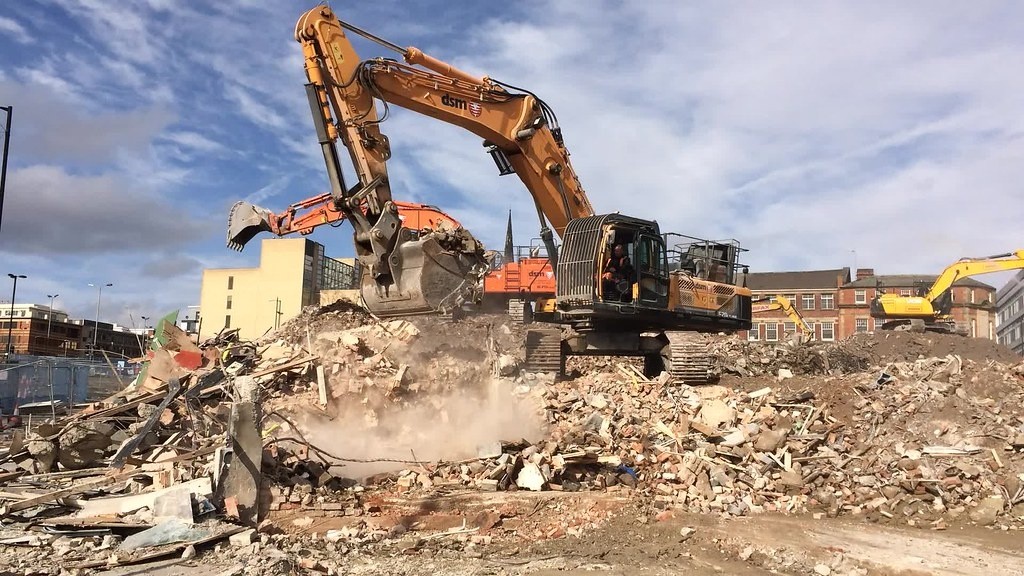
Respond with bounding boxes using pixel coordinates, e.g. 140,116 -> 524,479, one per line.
601,244 -> 630,302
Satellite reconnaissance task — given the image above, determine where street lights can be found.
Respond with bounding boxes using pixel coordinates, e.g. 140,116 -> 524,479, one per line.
47,294 -> 60,338
87,281 -> 113,347
145,325 -> 153,356
140,316 -> 150,358
5,273 -> 28,364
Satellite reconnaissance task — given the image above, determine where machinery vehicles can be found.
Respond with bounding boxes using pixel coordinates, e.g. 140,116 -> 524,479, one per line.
293,2 -> 755,389
867,246 -> 1024,337
224,189 -> 558,326
750,294 -> 816,346
